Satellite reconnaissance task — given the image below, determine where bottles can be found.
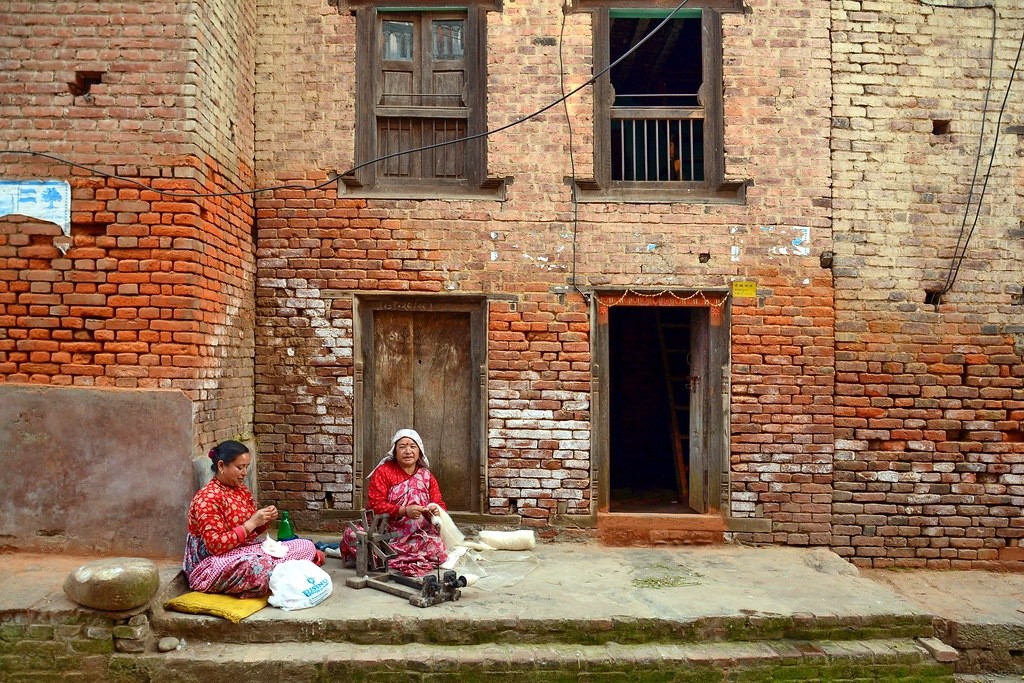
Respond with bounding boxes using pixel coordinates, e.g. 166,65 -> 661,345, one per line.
277,511 -> 295,542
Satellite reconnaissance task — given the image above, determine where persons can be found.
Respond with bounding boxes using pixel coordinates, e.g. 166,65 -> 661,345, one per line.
181,439 -> 326,599
365,429 -> 448,577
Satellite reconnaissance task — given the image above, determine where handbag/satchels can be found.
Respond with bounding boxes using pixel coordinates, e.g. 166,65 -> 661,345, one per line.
266,560 -> 333,613
